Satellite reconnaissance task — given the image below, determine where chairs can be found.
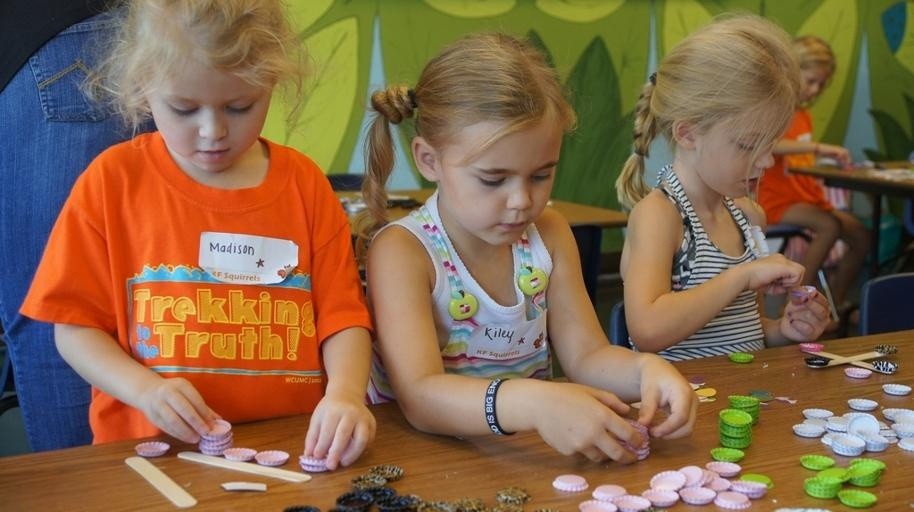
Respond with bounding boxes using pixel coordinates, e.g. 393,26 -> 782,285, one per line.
856,272 -> 914,335
609,301 -> 632,345
764,224 -> 839,322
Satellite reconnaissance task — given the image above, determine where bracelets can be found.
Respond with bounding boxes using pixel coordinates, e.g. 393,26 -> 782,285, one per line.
484,377 -> 516,436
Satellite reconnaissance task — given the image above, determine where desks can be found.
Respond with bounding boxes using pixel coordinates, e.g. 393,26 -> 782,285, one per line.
333,189 -> 629,229
0,329 -> 913,512
787,161 -> 914,265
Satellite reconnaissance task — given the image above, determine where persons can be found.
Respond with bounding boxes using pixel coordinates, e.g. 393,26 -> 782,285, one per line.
1,0 -> 159,451
347,31 -> 702,465
752,35 -> 868,312
615,12 -> 832,362
16,0 -> 378,472
